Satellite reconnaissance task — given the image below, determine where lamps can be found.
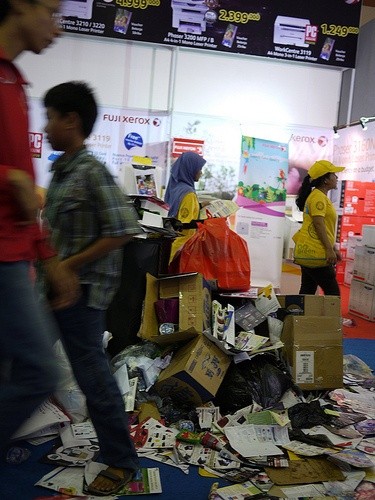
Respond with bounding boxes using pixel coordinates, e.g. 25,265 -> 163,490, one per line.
360,116 -> 375,130
333,126 -> 340,138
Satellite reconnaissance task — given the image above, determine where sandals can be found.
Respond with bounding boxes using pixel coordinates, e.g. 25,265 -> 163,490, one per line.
85,466 -> 137,495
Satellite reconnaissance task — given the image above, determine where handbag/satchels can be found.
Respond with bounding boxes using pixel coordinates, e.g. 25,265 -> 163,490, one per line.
293,236 -> 331,267
178,215 -> 251,292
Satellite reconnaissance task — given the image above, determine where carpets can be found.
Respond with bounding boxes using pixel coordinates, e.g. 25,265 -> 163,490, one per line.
333,279 -> 375,341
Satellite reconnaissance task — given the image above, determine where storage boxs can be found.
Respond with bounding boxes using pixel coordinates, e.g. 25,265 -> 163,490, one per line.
334,250 -> 346,286
330,178 -> 375,218
343,259 -> 353,287
348,280 -> 375,323
274,292 -> 343,316
346,235 -> 362,260
152,333 -> 231,406
286,314 -> 345,393
353,247 -> 375,287
138,269 -> 218,344
360,224 -> 375,248
334,215 -> 375,252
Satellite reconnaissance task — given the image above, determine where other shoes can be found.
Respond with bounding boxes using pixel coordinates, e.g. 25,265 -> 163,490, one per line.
342,318 -> 354,328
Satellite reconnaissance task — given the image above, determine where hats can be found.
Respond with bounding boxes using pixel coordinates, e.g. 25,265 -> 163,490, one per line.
308,162 -> 345,182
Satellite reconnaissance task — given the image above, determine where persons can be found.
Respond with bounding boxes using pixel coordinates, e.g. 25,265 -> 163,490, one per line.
164,151 -> 207,235
33,82 -> 144,494
293,161 -> 345,296
0,0 -> 79,449
